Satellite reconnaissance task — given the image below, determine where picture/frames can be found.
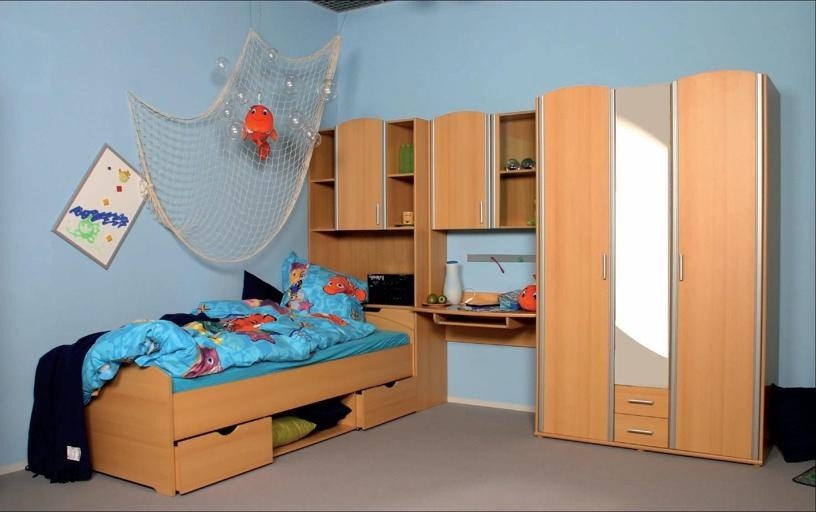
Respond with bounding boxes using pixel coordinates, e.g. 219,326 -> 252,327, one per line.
51,142 -> 152,271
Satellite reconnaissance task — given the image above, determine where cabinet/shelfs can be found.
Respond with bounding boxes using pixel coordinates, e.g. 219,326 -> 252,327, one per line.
429,96 -> 542,231
534,68 -> 780,466
307,116 -> 446,408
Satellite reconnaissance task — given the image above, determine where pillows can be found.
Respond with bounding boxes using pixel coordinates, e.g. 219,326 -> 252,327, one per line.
273,416 -> 317,448
289,399 -> 352,431
280,249 -> 367,321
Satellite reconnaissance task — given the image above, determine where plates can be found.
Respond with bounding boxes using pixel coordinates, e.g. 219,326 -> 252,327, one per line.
420,303 -> 452,308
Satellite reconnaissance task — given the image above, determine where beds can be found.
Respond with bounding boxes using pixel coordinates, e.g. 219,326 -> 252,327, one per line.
85,313 -> 417,496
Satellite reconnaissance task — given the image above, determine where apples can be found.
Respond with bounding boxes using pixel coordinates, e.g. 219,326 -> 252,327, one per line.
427,294 -> 438,304
437,295 -> 447,304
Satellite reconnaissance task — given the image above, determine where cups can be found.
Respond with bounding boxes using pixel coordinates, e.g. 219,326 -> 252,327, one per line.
400,211 -> 414,224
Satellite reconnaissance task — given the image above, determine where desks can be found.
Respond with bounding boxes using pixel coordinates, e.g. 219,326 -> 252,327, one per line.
415,294 -> 537,345
